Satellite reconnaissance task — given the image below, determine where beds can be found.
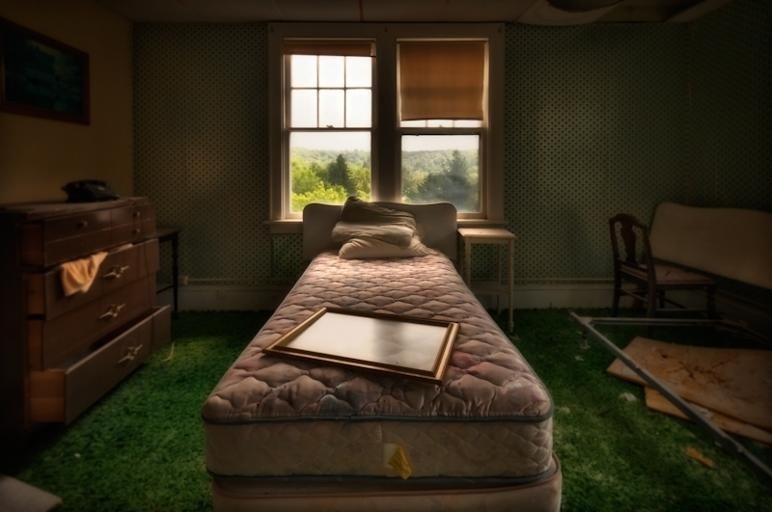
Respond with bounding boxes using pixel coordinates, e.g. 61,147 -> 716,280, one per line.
196,202 -> 566,512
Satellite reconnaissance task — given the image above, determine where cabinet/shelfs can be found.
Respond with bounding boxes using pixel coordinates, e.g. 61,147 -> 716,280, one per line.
0,197 -> 174,429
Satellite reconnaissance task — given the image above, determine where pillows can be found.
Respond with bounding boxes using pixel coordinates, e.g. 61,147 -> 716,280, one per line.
338,233 -> 439,261
328,195 -> 418,247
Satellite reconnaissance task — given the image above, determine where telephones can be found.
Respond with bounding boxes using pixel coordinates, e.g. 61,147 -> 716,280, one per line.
61,179 -> 121,200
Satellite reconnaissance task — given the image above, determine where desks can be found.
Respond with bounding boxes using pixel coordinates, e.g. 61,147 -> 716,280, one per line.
157,223 -> 180,319
457,226 -> 519,334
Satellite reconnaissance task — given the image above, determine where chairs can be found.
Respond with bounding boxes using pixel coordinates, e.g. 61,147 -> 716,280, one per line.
607,210 -> 719,331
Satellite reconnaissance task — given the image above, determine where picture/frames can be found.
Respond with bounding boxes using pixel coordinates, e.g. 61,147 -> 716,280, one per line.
0,14 -> 93,127
259,303 -> 460,385
